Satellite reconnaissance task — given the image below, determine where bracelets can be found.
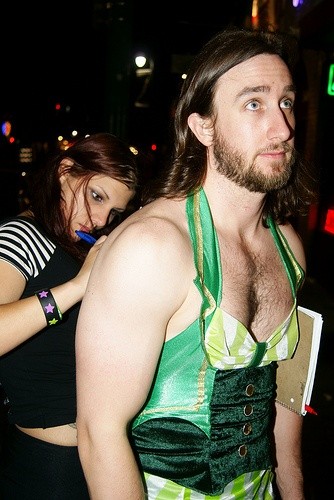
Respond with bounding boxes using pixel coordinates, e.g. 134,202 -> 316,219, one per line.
33,287 -> 63,329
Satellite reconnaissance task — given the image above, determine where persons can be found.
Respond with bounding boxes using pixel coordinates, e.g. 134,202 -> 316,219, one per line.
76,30 -> 310,499
0,134 -> 145,499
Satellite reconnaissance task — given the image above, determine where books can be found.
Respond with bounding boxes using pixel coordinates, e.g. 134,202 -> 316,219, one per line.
270,304 -> 324,418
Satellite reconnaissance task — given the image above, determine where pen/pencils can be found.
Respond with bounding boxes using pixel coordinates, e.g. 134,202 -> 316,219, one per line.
74,228 -> 97,245
303,404 -> 318,416
